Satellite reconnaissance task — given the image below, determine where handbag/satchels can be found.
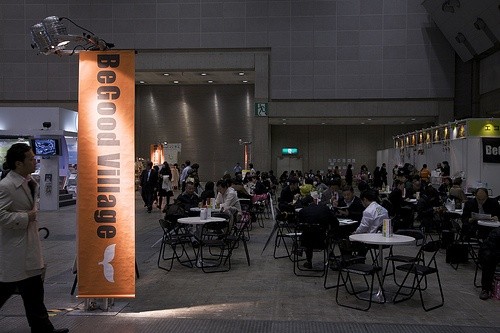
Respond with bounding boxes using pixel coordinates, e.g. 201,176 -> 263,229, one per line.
275,202 -> 295,223
446,246 -> 465,270
14,263 -> 46,294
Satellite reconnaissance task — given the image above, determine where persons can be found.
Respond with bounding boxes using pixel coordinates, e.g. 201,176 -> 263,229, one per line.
0,143 -> 69,333
139,159 -> 500,300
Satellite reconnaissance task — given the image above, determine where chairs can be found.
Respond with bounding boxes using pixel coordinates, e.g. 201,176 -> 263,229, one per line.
157,184 -> 483,312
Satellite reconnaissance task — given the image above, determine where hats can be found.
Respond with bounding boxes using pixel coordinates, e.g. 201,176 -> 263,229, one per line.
300,184 -> 311,195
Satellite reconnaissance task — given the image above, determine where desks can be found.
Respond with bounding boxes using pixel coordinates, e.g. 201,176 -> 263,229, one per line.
177,189 -> 500,303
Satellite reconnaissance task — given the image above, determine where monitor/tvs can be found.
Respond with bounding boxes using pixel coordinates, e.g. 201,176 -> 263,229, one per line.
32,138 -> 59,156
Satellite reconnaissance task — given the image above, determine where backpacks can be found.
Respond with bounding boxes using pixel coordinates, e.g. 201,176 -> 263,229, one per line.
162,199 -> 184,222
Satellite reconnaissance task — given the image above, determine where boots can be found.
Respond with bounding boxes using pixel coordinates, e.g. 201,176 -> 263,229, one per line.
157,198 -> 163,209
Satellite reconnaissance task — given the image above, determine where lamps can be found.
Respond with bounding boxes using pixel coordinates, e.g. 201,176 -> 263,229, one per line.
29,15 -> 71,57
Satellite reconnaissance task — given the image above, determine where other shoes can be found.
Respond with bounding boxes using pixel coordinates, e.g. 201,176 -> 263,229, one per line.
148,210 -> 151,213
303,262 -> 312,269
479,290 -> 488,300
291,248 -> 303,256
51,328 -> 69,333
